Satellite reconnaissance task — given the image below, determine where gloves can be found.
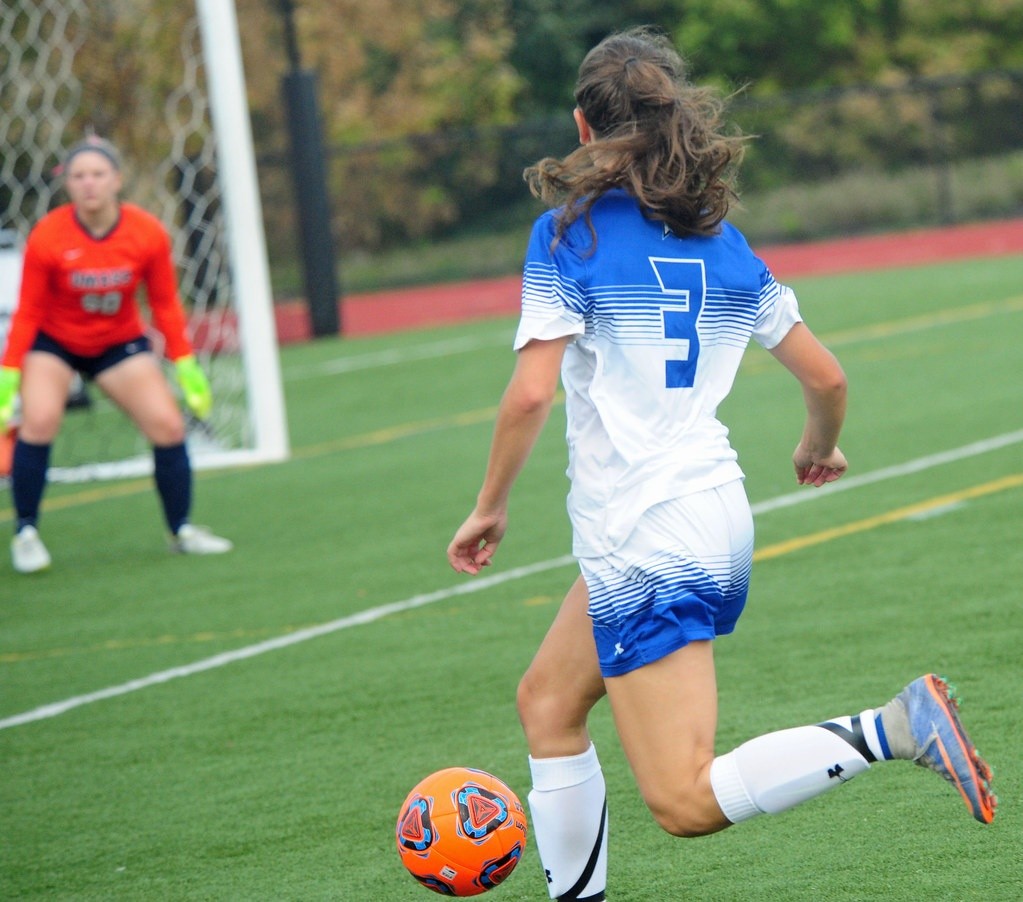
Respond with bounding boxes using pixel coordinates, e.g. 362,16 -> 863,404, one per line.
0,366 -> 19,433
174,353 -> 214,424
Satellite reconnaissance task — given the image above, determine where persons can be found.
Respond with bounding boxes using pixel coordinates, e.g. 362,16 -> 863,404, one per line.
445,31 -> 994,902
0,137 -> 232,573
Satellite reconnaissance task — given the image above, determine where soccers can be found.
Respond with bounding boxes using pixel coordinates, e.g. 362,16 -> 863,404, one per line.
394,766 -> 528,898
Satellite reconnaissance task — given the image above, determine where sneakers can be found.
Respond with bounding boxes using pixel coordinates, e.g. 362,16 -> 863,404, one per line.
169,523 -> 232,555
897,672 -> 998,824
8,524 -> 52,575
